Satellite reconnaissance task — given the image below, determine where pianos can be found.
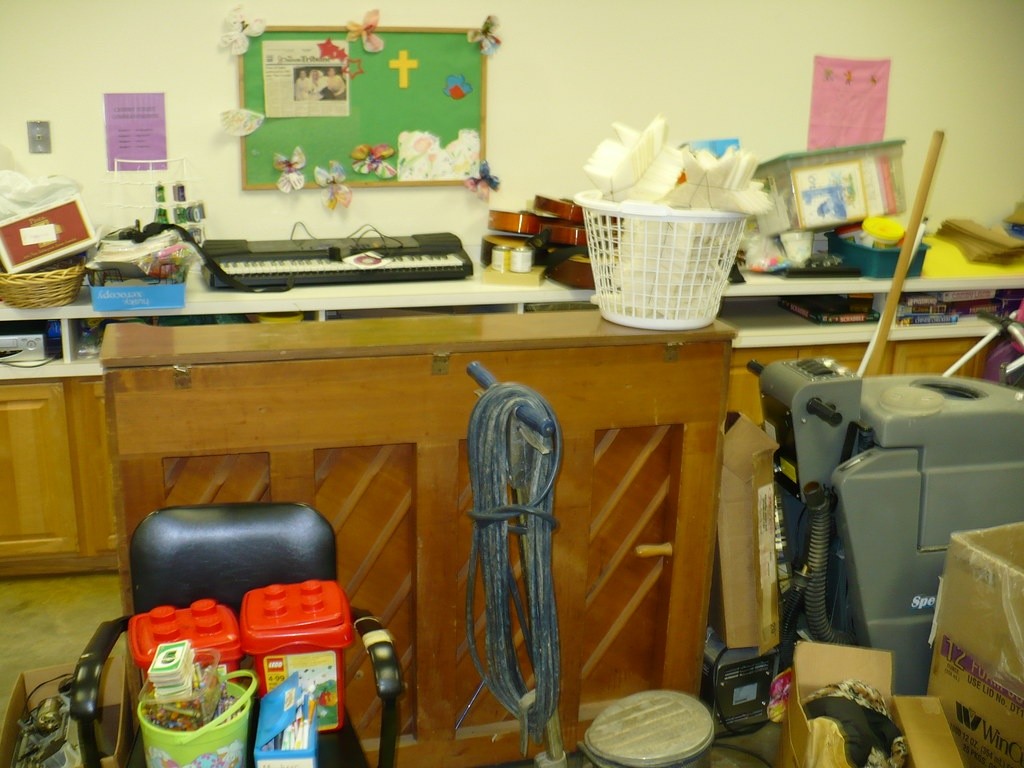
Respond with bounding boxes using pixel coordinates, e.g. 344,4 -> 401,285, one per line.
202,232 -> 475,288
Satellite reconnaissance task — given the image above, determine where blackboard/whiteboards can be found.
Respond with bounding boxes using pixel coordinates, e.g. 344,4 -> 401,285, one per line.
238,28 -> 488,192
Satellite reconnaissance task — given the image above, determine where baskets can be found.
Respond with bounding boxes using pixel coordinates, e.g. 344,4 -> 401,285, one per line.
572,190 -> 751,331
0,261 -> 88,309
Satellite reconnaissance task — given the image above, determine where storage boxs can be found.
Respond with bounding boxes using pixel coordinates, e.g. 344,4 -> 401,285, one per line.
822,233 -> 931,279
0,192 -> 98,274
778,640 -> 963,768
254,670 -> 318,768
927,522 -> 1024,768
716,365 -> 781,659
84,277 -> 186,311
756,139 -> 907,228
125,598 -> 242,682
0,652 -> 131,768
240,577 -> 356,733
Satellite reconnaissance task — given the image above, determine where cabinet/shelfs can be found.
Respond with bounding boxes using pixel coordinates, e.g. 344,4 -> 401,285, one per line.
0,274 -> 1024,768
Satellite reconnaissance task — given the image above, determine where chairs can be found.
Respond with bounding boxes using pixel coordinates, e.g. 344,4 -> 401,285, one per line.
68,500 -> 403,768
